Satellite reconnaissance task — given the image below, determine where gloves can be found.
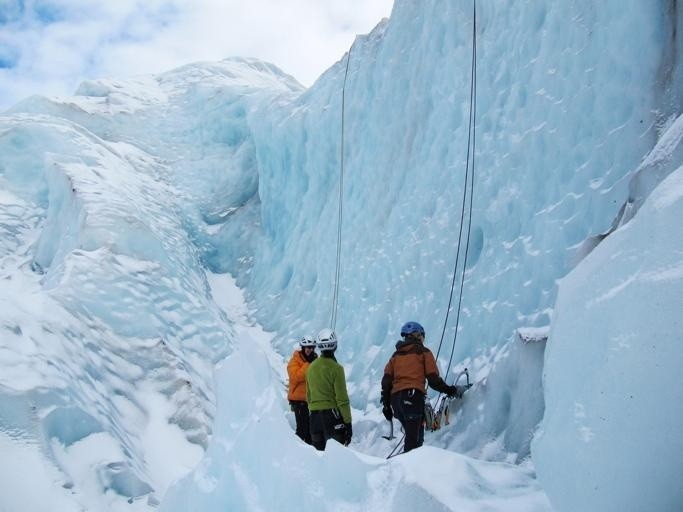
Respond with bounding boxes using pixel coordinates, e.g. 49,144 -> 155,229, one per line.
382,406 -> 394,420
346,423 -> 352,437
446,385 -> 457,397
307,351 -> 315,362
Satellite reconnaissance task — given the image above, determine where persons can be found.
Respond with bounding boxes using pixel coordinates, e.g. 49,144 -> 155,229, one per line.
378,322 -> 464,453
285,336 -> 318,445
303,328 -> 353,451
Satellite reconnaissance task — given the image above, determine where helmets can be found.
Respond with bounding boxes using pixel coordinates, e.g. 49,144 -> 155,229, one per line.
317,328 -> 337,351
400,322 -> 425,337
299,336 -> 316,347
